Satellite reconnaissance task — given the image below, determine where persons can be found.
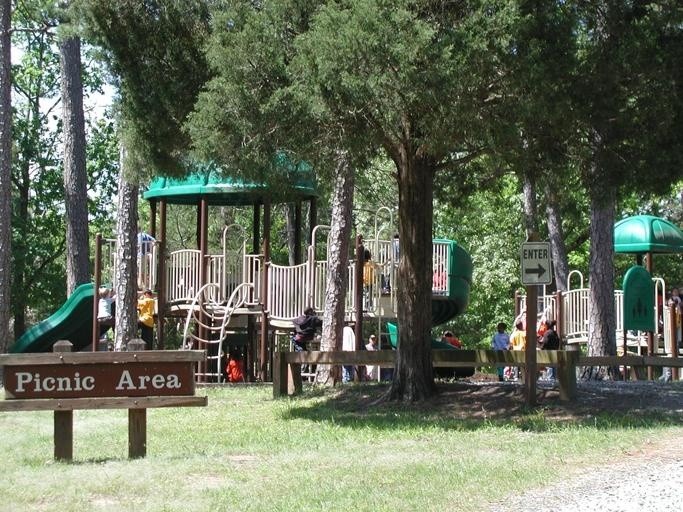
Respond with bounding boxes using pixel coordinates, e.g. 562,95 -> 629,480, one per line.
362,248 -> 389,311
340,319 -> 356,381
432,264 -> 447,292
289,306 -> 323,382
666,286 -> 682,349
538,318 -> 559,383
363,332 -> 379,382
656,286 -> 664,325
134,289 -> 157,350
224,353 -> 245,383
490,322 -> 511,383
95,286 -> 117,340
508,320 -> 526,385
440,330 -> 461,349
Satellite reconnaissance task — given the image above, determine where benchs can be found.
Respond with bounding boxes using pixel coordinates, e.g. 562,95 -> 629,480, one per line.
576,355 -> 683,381
273,350 -> 579,402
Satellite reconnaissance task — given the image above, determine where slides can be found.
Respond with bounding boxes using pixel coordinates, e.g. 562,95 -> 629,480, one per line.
7,283 -> 115,353
388,240 -> 476,378
